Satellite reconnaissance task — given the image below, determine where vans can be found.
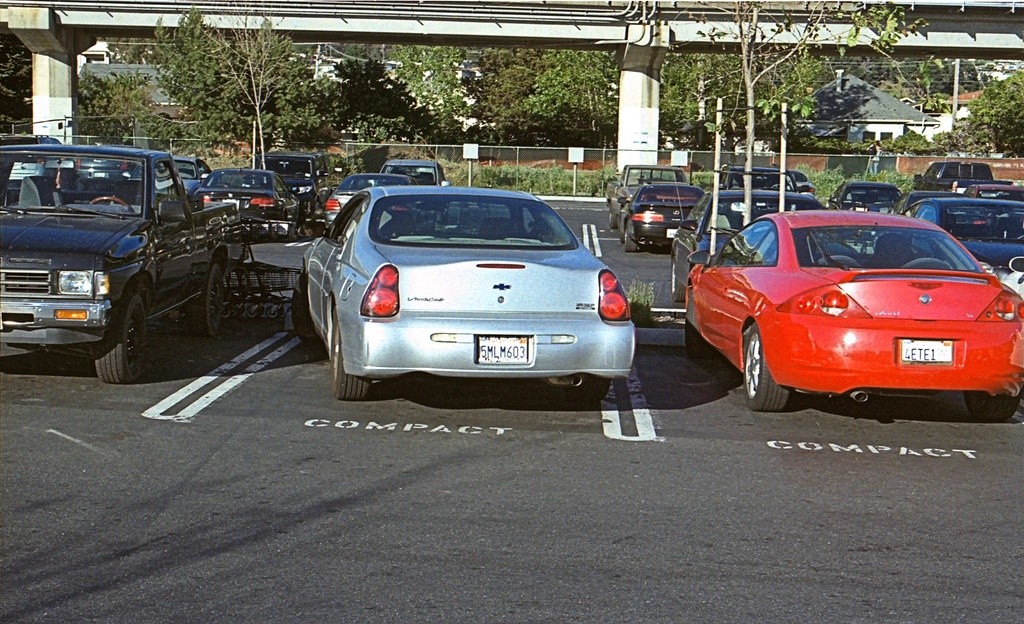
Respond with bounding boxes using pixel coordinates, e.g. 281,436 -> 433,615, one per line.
254,151 -> 332,219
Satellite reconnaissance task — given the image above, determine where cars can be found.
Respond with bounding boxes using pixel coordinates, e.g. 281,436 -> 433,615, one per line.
685,208 -> 1024,425
826,180 -> 903,215
617,183 -> 707,253
325,172 -> 420,231
672,190 -> 828,302
196,167 -> 301,242
378,161 -> 452,188
171,155 -> 211,202
901,184 -> 1024,300
290,185 -> 636,403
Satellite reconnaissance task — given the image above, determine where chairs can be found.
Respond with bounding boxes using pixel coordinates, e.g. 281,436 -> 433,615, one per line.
874,232 -> 912,263
381,209 -> 418,238
17,176 -> 61,209
478,216 -> 517,241
417,172 -> 435,184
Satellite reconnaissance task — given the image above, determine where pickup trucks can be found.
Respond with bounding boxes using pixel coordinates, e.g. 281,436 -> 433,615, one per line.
605,164 -> 688,229
914,161 -> 1014,194
0,143 -> 244,386
